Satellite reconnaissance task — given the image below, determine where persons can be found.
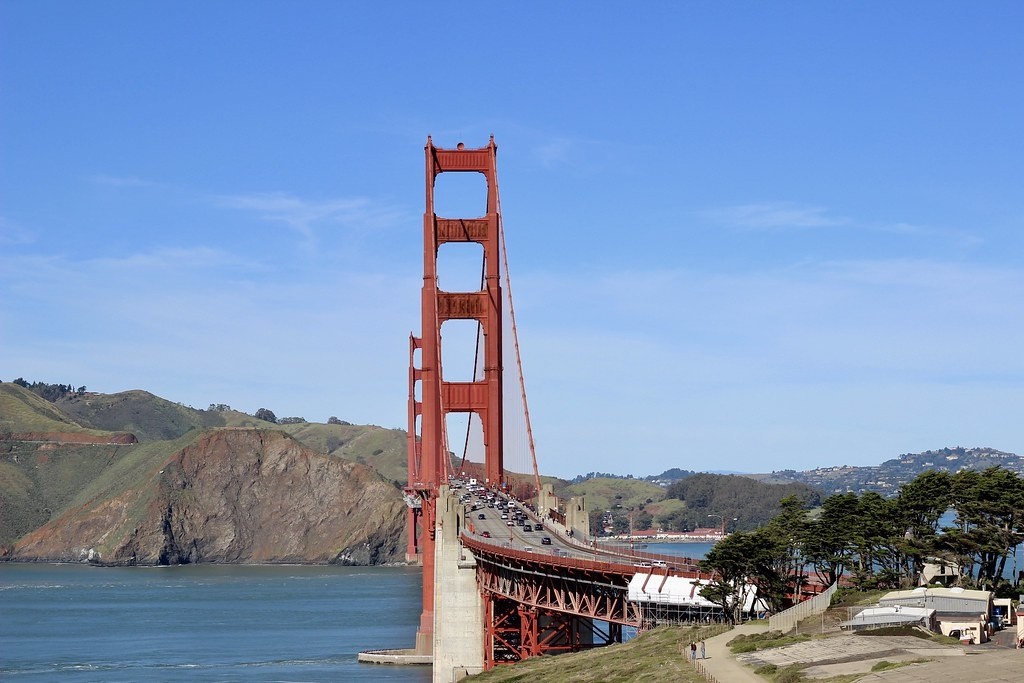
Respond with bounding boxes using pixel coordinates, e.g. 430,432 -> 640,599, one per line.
700,637 -> 705,660
682,556 -> 692,565
690,641 -> 696,660
563,527 -> 574,538
660,610 -> 767,625
590,540 -> 597,549
489,482 -> 558,524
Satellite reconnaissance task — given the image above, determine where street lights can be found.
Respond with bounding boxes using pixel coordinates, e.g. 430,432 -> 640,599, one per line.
617,504 -> 635,557
708,514 -> 725,538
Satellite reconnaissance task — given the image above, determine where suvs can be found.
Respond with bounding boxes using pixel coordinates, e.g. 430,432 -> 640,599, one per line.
507,501 -> 515,509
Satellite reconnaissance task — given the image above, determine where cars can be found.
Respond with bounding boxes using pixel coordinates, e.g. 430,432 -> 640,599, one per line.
482,531 -> 491,538
518,519 -> 525,526
448,474 -> 509,511
541,537 -> 551,544
535,523 -> 543,530
501,513 -> 508,519
516,509 -> 522,515
503,508 -> 509,513
512,507 -> 518,512
478,513 -> 484,520
550,547 -> 570,558
522,513 -> 528,520
508,520 -> 514,527
524,524 -> 532,532
511,515 -> 518,521
525,547 -> 534,554
634,559 -> 701,572
